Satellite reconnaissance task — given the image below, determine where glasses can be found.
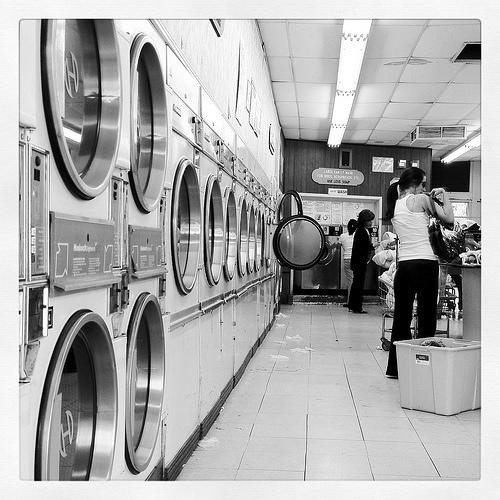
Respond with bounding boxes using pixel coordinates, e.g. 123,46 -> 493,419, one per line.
420,180 -> 428,188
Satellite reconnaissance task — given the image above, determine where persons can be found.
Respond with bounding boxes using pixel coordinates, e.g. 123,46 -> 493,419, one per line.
387,165 -> 453,379
334,209 -> 380,314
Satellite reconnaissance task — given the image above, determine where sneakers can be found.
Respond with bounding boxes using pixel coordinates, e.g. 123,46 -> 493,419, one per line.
348,308 -> 368,313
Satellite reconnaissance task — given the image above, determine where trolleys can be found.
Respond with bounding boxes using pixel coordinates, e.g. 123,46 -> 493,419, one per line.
377,249 -> 457,353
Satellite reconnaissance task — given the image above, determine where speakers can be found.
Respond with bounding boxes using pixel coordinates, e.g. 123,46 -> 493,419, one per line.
339,148 -> 353,169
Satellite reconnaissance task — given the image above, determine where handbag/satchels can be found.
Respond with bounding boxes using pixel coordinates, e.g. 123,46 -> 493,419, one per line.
428,224 -> 466,262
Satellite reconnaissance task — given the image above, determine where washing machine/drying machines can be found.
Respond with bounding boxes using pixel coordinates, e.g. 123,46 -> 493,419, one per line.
20,19 -> 342,481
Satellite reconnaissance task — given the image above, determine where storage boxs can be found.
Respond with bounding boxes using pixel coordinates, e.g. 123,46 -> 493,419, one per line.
392,333 -> 480,417
